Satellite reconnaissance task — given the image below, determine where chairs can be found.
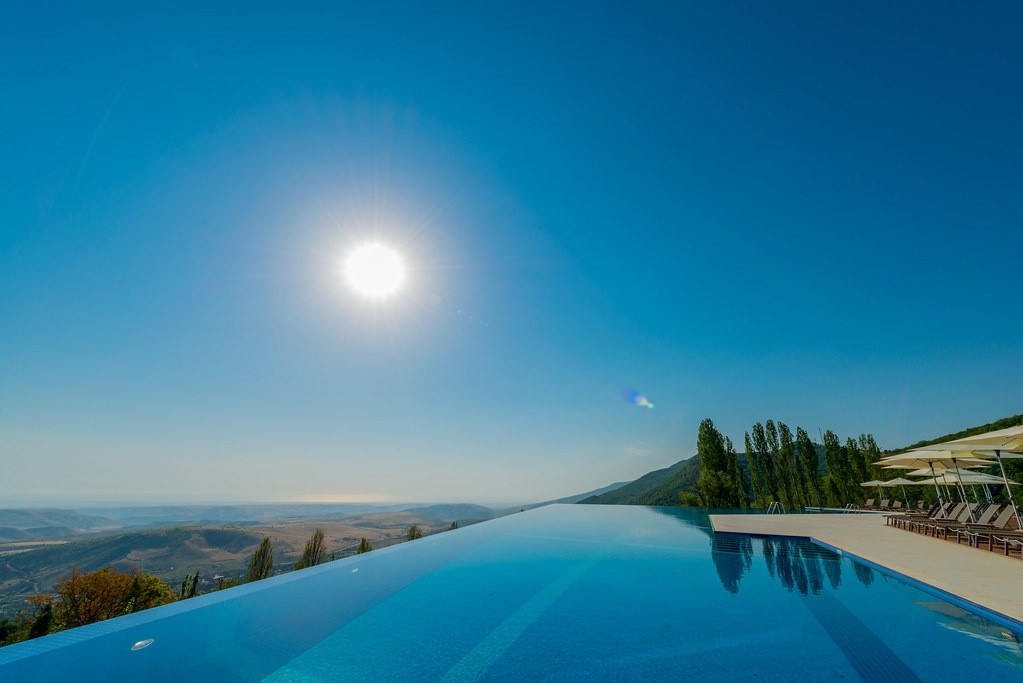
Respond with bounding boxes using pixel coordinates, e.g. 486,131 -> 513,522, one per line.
859,499 -> 1023,555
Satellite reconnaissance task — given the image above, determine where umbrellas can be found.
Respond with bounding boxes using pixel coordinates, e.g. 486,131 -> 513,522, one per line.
877,477 -> 916,508
860,480 -> 896,501
874,426 -> 1023,530
